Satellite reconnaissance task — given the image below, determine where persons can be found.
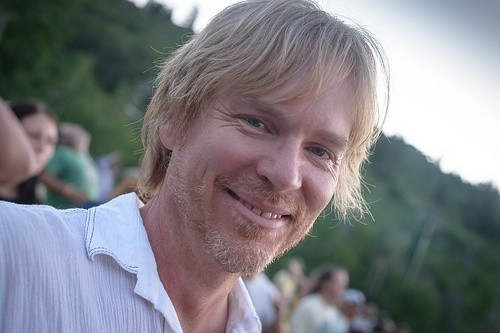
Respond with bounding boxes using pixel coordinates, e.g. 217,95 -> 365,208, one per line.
0,0 -> 382,333
1,90 -> 399,333
